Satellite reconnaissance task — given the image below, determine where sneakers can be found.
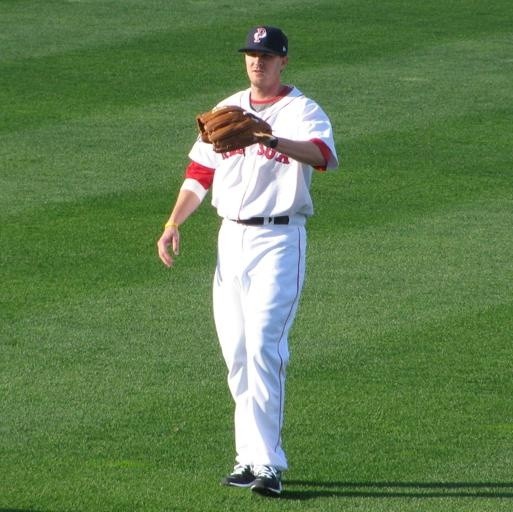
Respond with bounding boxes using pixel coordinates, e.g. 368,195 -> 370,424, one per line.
250,464 -> 284,495
221,464 -> 257,489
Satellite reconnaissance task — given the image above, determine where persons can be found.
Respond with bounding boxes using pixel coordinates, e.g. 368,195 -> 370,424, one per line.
156,25 -> 339,496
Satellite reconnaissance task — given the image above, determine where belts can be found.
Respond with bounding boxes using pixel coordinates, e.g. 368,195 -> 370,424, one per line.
225,213 -> 292,224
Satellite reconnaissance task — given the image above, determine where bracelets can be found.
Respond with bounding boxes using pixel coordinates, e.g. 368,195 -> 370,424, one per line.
163,222 -> 178,229
265,135 -> 277,149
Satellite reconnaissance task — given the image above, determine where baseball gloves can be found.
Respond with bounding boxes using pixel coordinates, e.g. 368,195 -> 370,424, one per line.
195,105 -> 271,153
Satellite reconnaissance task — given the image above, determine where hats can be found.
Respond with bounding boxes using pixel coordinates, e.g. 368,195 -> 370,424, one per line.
237,25 -> 289,58
